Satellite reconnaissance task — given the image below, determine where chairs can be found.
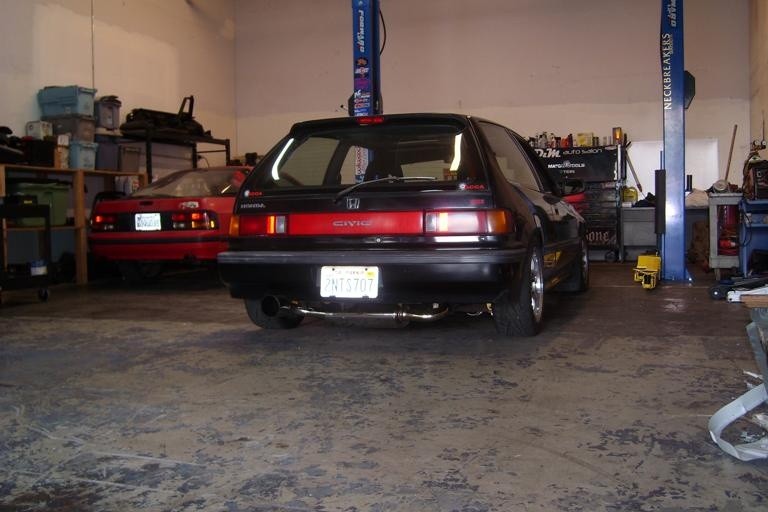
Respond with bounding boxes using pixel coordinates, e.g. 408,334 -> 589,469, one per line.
361,158 -> 404,184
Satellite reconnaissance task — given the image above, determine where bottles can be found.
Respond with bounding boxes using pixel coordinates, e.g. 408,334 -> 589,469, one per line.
529,131 -> 573,148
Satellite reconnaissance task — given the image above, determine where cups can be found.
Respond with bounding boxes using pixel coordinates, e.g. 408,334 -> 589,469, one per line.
604,136 -> 611,146
592,137 -> 599,146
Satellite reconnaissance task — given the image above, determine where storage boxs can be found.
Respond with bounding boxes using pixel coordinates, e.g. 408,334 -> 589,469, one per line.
24,84 -> 196,185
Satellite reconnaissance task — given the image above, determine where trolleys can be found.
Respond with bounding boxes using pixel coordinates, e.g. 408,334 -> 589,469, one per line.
0,193 -> 55,303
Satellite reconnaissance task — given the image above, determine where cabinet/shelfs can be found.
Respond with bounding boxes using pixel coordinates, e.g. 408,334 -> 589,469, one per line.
621,190 -> 768,283
0,161 -> 150,291
96,132 -> 231,193
527,142 -> 624,266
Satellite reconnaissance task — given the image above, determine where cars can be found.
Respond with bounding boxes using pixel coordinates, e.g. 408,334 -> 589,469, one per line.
215,115 -> 595,345
96,165 -> 312,285
